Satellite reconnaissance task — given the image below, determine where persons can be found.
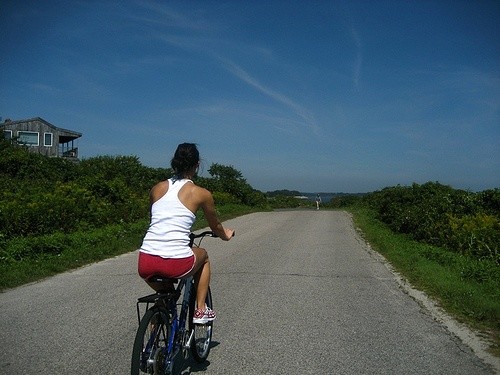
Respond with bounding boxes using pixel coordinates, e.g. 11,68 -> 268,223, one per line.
137,143 -> 236,345
315,193 -> 321,207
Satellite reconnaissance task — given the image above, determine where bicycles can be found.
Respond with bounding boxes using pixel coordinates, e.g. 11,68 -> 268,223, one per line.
129,229 -> 236,375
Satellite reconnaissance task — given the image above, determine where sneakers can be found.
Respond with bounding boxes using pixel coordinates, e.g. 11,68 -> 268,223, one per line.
193,302 -> 216,324
